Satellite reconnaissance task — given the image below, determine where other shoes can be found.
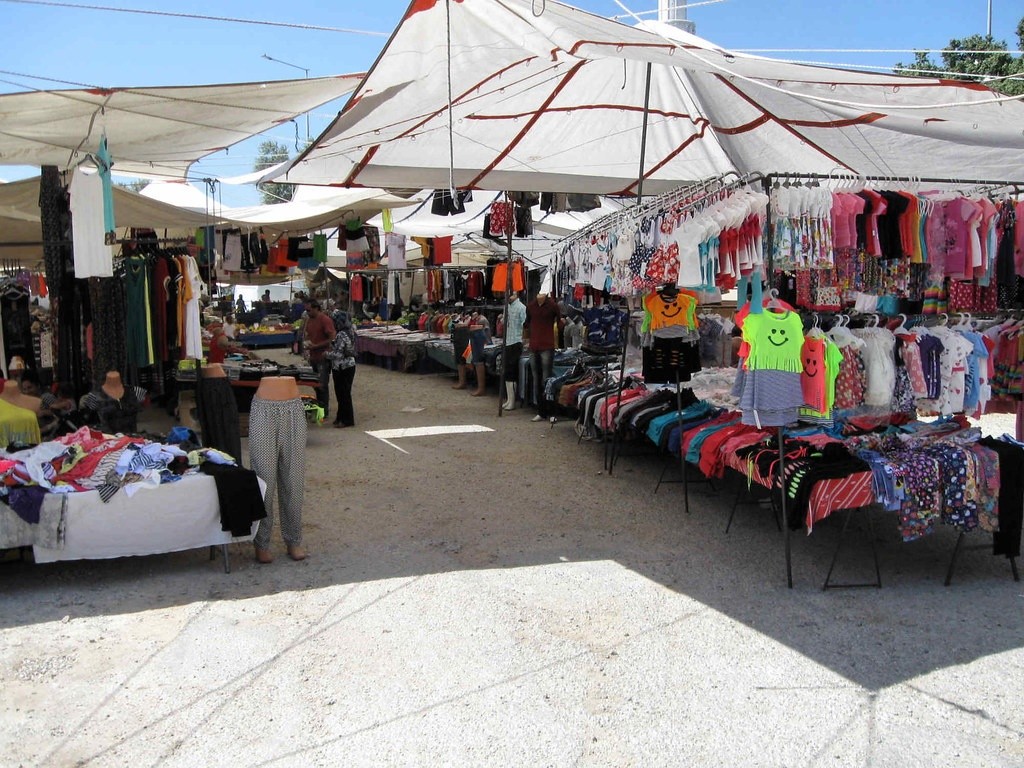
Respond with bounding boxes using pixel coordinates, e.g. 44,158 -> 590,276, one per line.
332,421 -> 354,428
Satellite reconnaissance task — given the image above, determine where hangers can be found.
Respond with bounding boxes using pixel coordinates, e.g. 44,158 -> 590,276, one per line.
762,280 -> 786,310
0,257 -> 22,299
110,236 -> 199,265
48,128 -> 114,179
550,170 -> 1024,260
794,307 -> 1024,338
492,199 -> 512,204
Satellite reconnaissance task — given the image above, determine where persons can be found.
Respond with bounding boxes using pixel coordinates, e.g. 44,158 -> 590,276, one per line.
554,317 -> 583,349
236,294 -> 248,315
201,363 -> 242,466
200,300 -> 205,327
325,297 -> 340,316
219,296 -> 228,313
528,294 -> 564,423
0,380 -> 42,414
79,372 -> 147,433
9,356 -> 25,370
248,377 -> 307,562
496,294 -> 527,410
368,297 -> 381,313
282,299 -> 337,422
29,308 -> 58,345
418,310 -> 491,396
208,328 -> 228,363
731,327 -> 743,367
390,298 -> 403,321
323,313 -> 356,427
223,315 -> 237,341
261,289 -> 272,304
294,290 -> 308,303
0,368 -> 4,394
409,297 -> 423,312
21,378 -> 78,439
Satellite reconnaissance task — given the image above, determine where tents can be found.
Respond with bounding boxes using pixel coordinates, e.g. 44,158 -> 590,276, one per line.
0,0 -> 1024,271
